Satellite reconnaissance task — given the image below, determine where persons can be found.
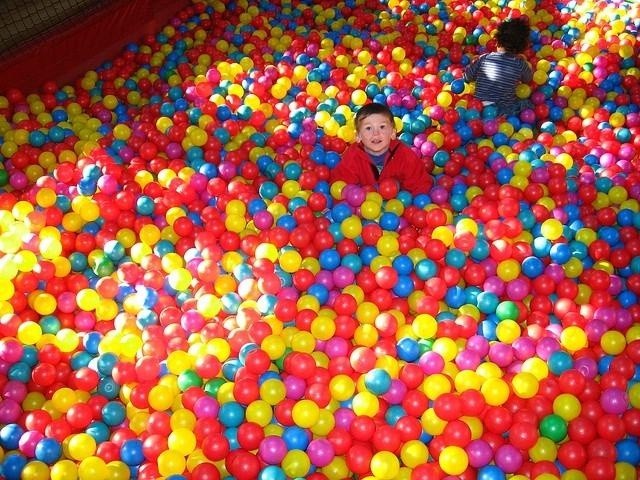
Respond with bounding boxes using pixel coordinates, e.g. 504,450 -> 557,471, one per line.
463,17 -> 533,120
328,103 -> 433,205
